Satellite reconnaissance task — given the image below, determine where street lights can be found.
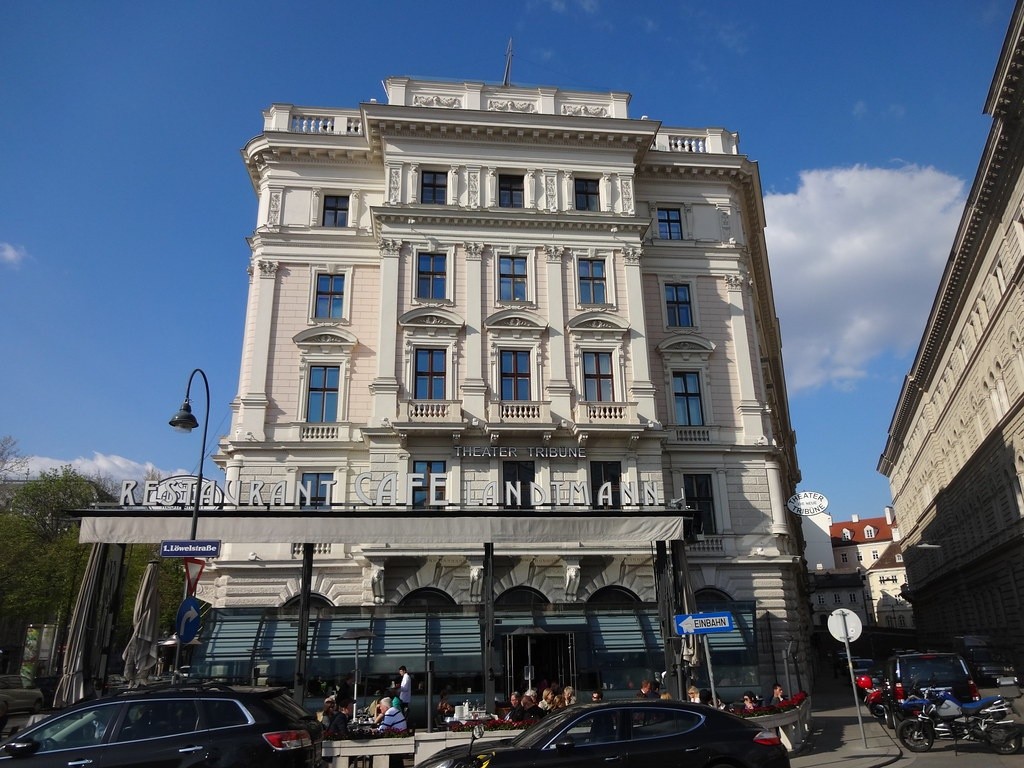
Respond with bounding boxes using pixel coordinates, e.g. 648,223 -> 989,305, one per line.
169,368 -> 211,674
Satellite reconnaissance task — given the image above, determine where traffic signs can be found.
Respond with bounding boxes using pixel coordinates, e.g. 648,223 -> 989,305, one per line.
673,611 -> 734,636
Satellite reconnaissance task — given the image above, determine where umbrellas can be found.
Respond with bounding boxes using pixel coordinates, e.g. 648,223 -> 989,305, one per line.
680,541 -> 704,667
120,559 -> 160,688
52,543 -> 103,708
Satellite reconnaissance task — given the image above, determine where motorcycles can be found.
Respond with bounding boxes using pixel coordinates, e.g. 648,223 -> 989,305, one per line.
896,670 -> 1024,757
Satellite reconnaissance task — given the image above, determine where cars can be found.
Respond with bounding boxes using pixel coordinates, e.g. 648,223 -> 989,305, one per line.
0,675 -> 45,718
833,646 -> 1024,737
411,696 -> 791,768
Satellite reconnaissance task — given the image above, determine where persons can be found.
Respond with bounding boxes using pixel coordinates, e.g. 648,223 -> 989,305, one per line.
771,684 -> 786,705
88,678 -> 108,701
503,686 -> 576,722
369,689 -> 407,733
437,690 -> 455,714
591,690 -> 603,701
336,673 -> 353,706
744,691 -> 759,709
321,694 -> 357,731
636,680 -> 672,699
688,685 -> 728,708
397,666 -> 411,707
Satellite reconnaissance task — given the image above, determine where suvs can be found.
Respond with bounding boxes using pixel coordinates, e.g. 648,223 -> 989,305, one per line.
0,673 -> 324,768
